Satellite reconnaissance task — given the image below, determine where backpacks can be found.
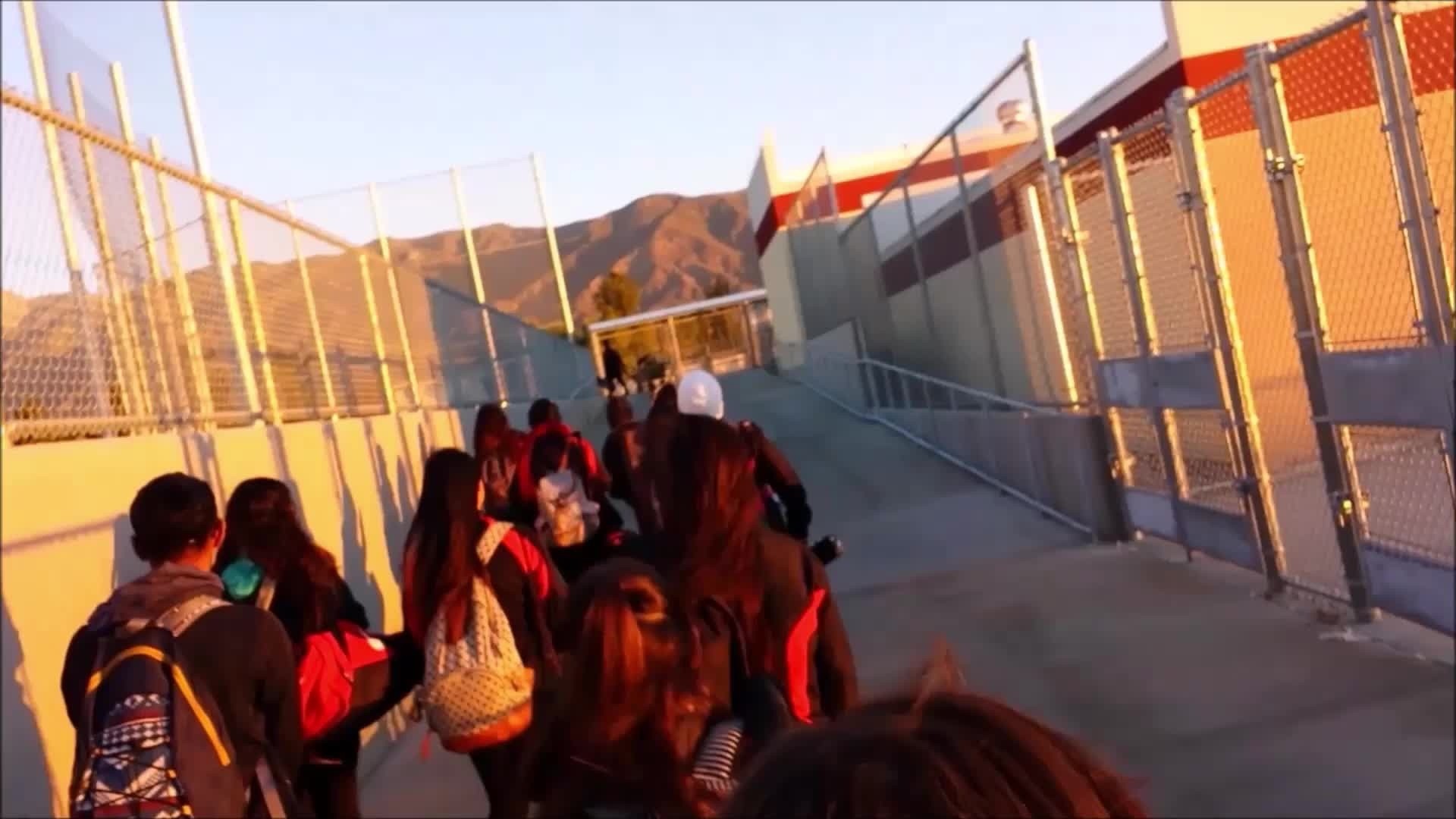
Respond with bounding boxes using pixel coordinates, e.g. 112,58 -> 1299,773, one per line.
535,469 -> 601,549
68,593 -> 248,818
416,522 -> 532,759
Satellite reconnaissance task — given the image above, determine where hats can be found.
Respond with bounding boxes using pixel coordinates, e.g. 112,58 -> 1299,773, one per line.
676,370 -> 725,421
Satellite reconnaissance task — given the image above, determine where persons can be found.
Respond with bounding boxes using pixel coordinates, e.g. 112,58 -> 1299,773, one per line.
600,339 -> 630,397
60,369 -> 864,819
707,678 -> 1152,819
